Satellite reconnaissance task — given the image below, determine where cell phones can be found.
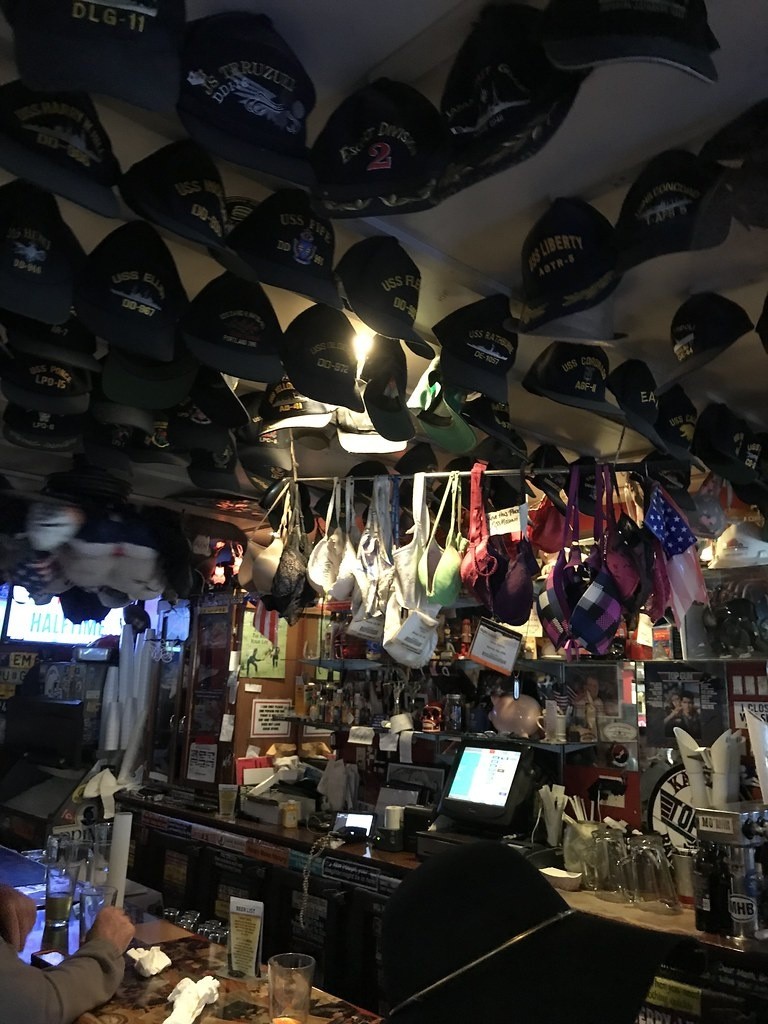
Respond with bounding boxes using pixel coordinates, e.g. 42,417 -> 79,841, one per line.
30,949 -> 69,968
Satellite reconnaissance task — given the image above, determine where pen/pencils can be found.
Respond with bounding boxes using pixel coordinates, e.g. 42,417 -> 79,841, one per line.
110,889 -> 119,907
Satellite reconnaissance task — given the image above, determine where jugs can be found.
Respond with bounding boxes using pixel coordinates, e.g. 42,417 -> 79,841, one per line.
564,822 -> 610,892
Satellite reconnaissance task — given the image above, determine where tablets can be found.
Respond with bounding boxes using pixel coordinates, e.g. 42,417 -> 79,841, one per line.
330,811 -> 377,839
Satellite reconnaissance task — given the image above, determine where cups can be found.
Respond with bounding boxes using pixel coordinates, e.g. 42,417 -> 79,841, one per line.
139,628 -> 155,712
269,954 -> 315,1024
119,697 -> 135,750
45,823 -> 113,885
134,635 -> 144,697
79,886 -> 118,946
580,829 -> 630,904
45,863 -> 80,927
616,835 -> 682,914
219,784 -> 238,817
385,806 -> 400,830
100,667 -> 119,750
537,715 -> 567,744
119,625 -> 134,700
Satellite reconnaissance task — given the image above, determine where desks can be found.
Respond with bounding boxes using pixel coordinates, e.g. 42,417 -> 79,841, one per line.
112,786 -> 767,960
0,851 -> 387,1024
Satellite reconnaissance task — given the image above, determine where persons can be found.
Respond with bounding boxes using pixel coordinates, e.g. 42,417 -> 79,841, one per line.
576,677 -> 602,705
663,691 -> 701,738
0,883 -> 135,1024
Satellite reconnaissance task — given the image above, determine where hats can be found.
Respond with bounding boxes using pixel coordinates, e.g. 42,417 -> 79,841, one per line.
0,1 -> 768,532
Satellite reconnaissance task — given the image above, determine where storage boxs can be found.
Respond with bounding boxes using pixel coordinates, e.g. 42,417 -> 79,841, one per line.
123,838 -> 386,1009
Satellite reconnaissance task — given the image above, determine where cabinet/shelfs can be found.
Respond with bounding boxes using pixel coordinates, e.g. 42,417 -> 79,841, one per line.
274,655 -> 598,778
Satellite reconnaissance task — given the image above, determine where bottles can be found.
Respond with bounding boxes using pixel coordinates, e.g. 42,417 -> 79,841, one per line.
283,800 -> 298,829
443,694 -> 462,735
304,683 -> 343,726
21,849 -> 45,865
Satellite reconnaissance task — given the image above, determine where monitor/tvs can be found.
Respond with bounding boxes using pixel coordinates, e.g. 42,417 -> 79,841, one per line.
436,739 -> 535,834
